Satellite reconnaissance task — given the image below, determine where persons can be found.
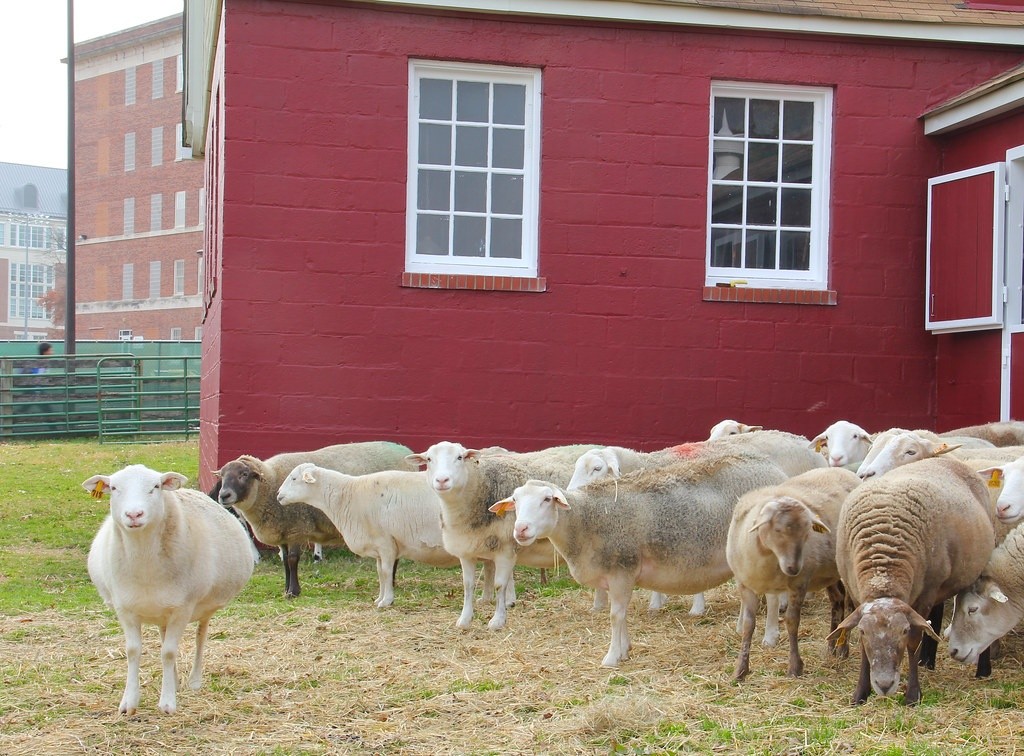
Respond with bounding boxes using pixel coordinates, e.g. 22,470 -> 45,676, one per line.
12,343 -> 59,431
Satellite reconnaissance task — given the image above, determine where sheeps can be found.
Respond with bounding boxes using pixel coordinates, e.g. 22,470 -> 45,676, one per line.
208,441 -> 420,599
276,462 -> 516,609
403,440 -> 608,633
81,465 -> 254,714
489,419 -> 1024,708
